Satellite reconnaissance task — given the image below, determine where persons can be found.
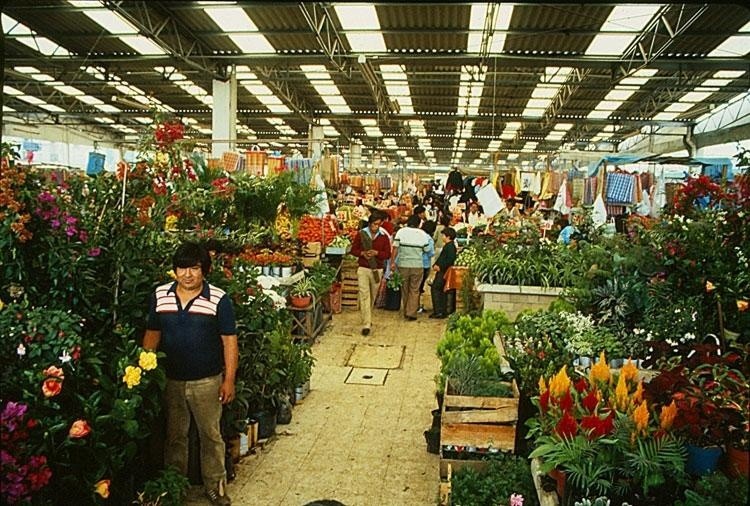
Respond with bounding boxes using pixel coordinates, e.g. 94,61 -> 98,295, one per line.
380,176 -> 453,313
428,225 -> 458,319
550,211 -> 584,245
141,238 -> 239,505
506,198 -> 519,216
461,203 -> 489,223
391,215 -> 432,321
350,212 -> 390,335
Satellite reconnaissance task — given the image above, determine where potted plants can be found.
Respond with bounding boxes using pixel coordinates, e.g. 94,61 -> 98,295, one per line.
440,338 -> 521,452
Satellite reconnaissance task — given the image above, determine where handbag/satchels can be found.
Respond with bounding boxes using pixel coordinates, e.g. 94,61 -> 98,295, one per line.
384,279 -> 401,310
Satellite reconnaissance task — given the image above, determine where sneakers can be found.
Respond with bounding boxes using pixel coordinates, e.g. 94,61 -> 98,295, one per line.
404,308 -> 448,320
362,328 -> 370,334
205,488 -> 231,506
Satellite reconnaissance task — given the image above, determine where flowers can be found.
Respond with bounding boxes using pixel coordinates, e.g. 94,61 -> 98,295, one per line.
451,168 -> 750,506
0,110 -> 342,506
